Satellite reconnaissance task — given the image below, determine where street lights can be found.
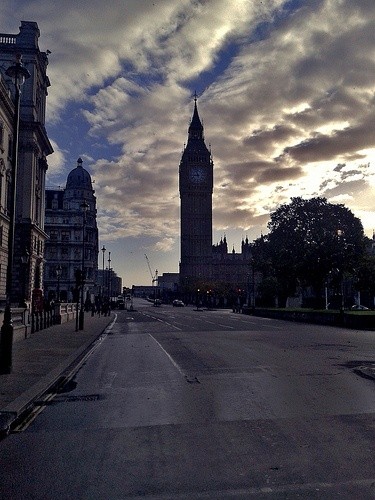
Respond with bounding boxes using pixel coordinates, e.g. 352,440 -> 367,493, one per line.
56,265 -> 62,302
20,248 -> 30,308
100,246 -> 107,314
79,200 -> 89,330
0,52 -> 30,378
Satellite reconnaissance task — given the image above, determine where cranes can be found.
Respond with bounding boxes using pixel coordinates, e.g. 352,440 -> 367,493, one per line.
145,254 -> 158,286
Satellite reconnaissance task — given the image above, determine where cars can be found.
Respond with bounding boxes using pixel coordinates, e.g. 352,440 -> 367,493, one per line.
173,300 -> 184,307
353,305 -> 368,310
